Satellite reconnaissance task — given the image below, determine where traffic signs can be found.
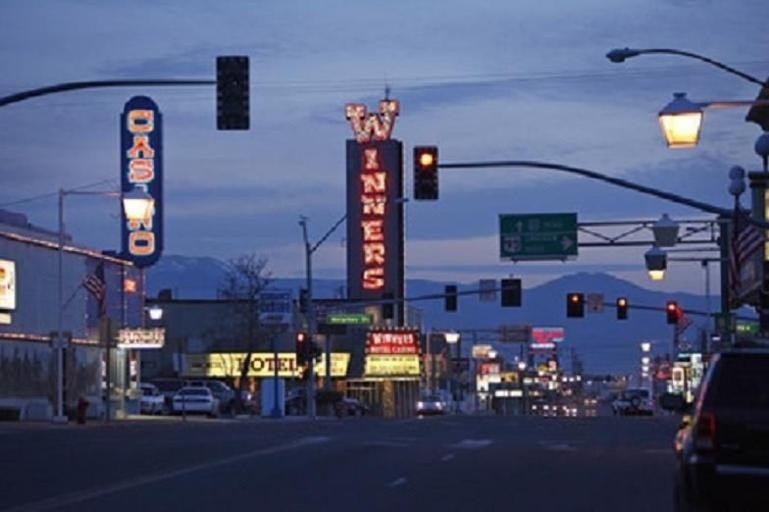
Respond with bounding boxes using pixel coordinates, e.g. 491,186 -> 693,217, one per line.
321,310 -> 374,326
500,212 -> 578,257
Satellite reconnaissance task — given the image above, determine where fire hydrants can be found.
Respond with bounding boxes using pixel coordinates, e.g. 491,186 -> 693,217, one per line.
76,392 -> 89,423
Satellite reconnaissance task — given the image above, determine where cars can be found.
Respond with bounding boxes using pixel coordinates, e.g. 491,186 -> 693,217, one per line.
531,388 -> 598,417
140,376 -> 369,418
415,396 -> 446,414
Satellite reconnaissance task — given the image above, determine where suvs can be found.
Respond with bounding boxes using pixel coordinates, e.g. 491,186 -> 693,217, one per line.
612,386 -> 653,416
661,340 -> 769,511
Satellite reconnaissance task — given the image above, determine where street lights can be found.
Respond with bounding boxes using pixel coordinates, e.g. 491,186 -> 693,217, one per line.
424,328 -> 462,397
643,246 -> 714,353
55,187 -> 154,424
652,210 -> 732,349
606,45 -> 768,90
656,89 -> 769,150
298,196 -> 410,421
105,304 -> 170,422
729,133 -> 769,231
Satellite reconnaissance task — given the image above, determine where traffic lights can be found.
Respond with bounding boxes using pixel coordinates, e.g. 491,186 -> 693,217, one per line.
667,301 -> 678,323
566,294 -> 583,316
301,287 -> 310,315
415,145 -> 439,198
617,297 -> 628,319
295,331 -> 309,344
